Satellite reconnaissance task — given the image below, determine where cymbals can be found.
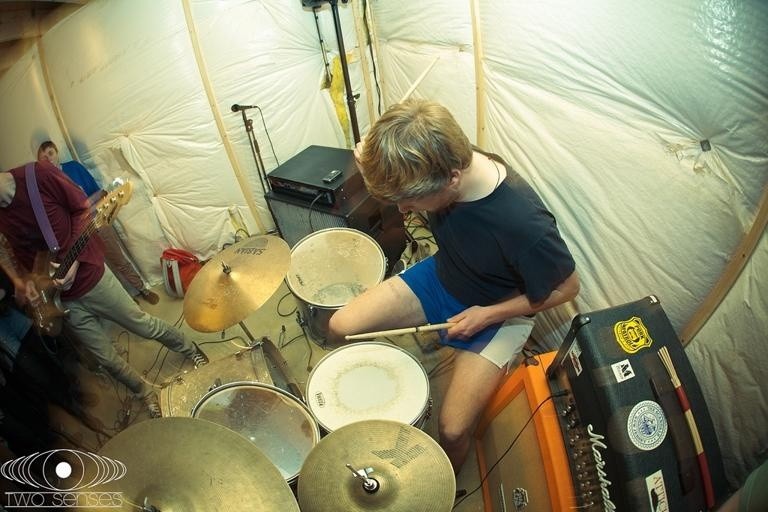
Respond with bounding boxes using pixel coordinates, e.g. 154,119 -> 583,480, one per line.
73,418 -> 300,512
183,235 -> 290,334
298,421 -> 455,512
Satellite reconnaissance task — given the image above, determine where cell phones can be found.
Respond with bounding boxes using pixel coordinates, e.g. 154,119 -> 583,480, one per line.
321,170 -> 342,184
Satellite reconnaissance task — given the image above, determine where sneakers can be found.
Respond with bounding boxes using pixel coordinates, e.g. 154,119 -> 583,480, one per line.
185,341 -> 210,369
141,288 -> 160,305
142,385 -> 163,419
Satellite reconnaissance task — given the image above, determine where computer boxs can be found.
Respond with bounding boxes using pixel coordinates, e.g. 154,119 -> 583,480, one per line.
263,190 -> 408,278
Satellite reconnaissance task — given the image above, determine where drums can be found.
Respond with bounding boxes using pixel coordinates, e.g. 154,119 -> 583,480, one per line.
192,382 -> 320,485
308,343 -> 430,430
285,229 -> 385,351
159,336 -> 307,418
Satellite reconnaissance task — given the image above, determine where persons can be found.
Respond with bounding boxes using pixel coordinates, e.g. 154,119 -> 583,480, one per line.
33,140 -> 163,303
0,163 -> 208,421
329,99 -> 580,475
1,308 -> 107,457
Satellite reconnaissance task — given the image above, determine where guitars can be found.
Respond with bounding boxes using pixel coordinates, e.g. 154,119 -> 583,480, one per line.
13,180 -> 134,337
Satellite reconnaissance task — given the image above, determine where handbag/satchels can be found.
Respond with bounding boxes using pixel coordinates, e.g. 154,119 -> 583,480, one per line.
160,247 -> 202,301
